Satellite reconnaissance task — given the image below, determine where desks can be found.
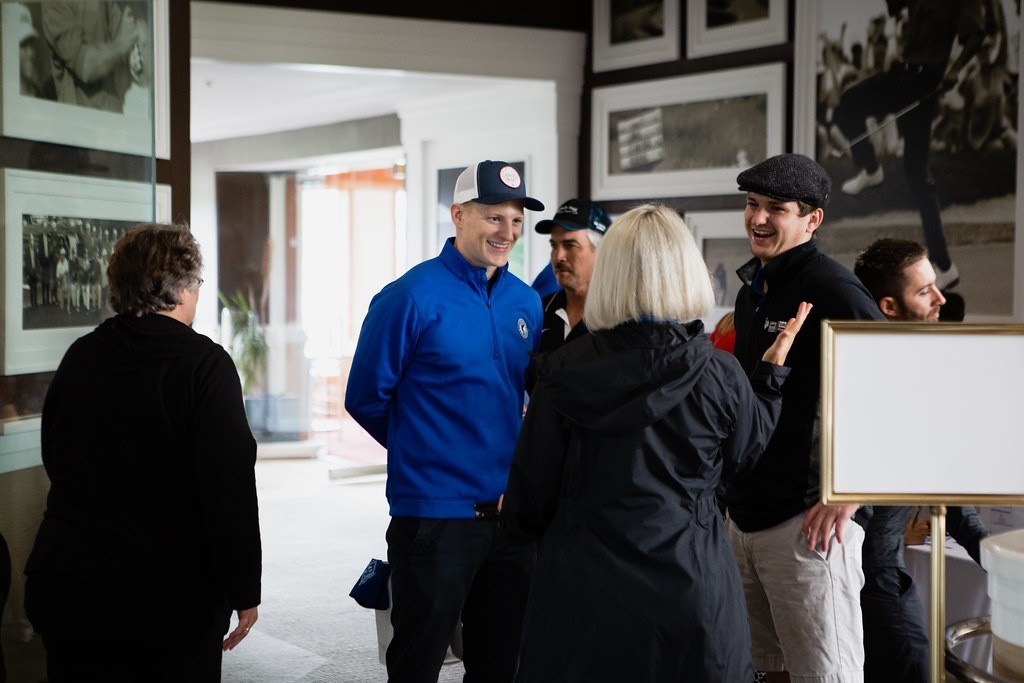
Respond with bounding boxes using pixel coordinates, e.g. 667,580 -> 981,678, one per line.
905,537 -> 994,683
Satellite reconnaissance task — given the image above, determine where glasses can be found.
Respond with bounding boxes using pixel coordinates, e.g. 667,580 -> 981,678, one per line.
190,275 -> 204,287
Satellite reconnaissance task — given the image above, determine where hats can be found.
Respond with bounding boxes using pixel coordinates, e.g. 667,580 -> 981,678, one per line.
535,198 -> 612,236
737,153 -> 831,210
453,160 -> 545,212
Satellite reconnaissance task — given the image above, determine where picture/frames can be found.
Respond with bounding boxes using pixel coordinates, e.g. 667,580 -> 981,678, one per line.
0,0 -> 171,159
0,167 -> 171,376
589,63 -> 787,202
793,0 -> 1024,325
591,0 -> 680,71
684,0 -> 789,60
684,209 -> 756,333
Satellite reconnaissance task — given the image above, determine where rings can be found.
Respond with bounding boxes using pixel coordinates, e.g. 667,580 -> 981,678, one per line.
245,628 -> 249,631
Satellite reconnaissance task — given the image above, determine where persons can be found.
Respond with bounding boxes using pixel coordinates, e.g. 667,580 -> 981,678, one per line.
850,236 -> 992,683
498,199 -> 814,683
18,0 -> 150,115
724,153 -> 887,683
23,214 -> 127,314
343,160 -> 543,683
23,222 -> 263,683
816,0 -> 1017,290
